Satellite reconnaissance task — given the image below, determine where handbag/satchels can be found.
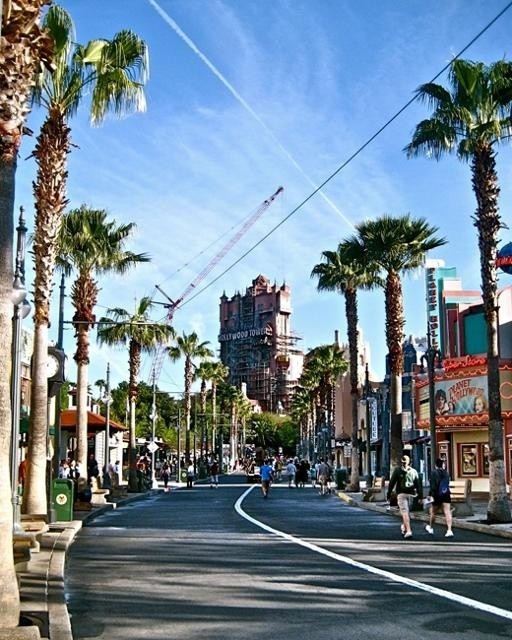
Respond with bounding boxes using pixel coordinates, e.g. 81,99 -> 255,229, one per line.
389,492 -> 398,506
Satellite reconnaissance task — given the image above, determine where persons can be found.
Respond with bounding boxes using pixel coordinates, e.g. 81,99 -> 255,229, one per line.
137,449 -> 335,497
425,458 -> 455,538
88,453 -> 99,485
113,460 -> 120,485
434,389 -> 456,415
58,451 -> 82,502
18,454 -> 29,495
103,459 -> 115,478
474,395 -> 488,414
385,455 -> 423,539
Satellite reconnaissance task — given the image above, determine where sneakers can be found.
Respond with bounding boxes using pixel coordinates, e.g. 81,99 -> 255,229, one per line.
444,531 -> 454,537
423,522 -> 434,535
400,523 -> 412,538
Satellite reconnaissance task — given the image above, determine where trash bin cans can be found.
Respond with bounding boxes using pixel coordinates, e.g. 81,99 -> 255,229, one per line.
127,470 -> 143,493
335,469 -> 347,490
50,478 -> 74,522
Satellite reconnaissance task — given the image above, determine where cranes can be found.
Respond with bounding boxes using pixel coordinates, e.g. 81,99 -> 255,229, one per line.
147,183 -> 288,386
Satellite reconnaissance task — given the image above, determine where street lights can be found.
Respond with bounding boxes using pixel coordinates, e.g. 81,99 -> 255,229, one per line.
321,421 -> 329,462
101,361 -> 114,475
11,201 -> 32,530
415,319 -> 447,479
148,366 -> 160,490
317,430 -> 323,458
359,362 -> 380,490
